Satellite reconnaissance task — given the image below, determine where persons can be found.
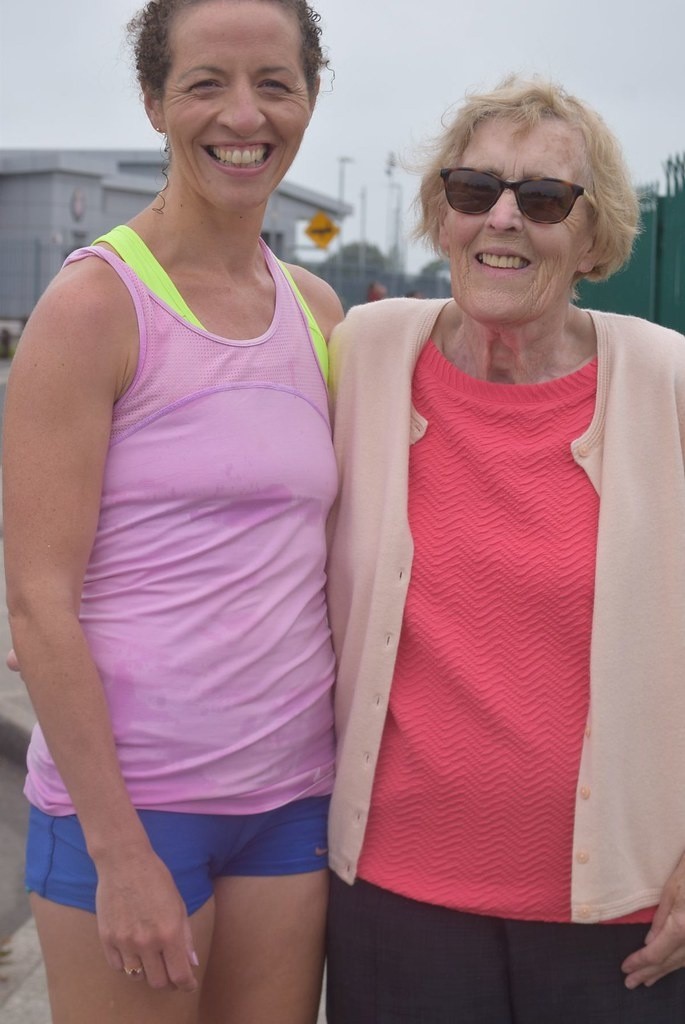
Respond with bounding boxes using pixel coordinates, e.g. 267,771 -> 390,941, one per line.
366,278 -> 423,300
0,0 -> 341,1024
323,86 -> 685,1023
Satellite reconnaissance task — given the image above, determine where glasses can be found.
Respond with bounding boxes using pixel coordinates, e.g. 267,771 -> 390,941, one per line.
440,167 -> 598,224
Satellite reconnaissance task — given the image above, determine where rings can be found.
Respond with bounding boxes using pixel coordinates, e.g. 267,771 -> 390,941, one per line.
123,967 -> 143,975
